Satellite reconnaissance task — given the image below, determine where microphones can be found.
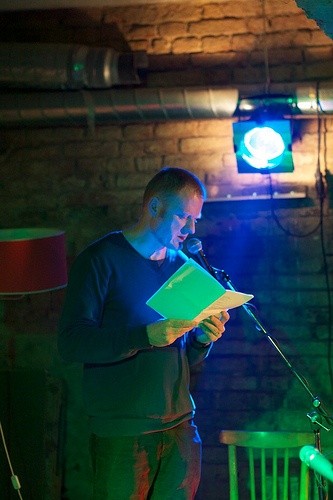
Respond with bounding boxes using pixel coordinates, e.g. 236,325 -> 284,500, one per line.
186,238 -> 215,276
298,445 -> 333,481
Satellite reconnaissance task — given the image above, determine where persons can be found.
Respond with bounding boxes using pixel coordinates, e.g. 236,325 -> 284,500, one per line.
58,167 -> 231,500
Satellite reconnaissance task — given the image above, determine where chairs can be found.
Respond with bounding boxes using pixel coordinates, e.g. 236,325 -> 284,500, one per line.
219,430 -> 316,500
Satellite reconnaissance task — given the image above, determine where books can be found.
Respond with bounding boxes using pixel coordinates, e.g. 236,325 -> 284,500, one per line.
145,257 -> 254,328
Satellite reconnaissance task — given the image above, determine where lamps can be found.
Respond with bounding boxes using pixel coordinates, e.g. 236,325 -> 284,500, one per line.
231,108 -> 297,174
